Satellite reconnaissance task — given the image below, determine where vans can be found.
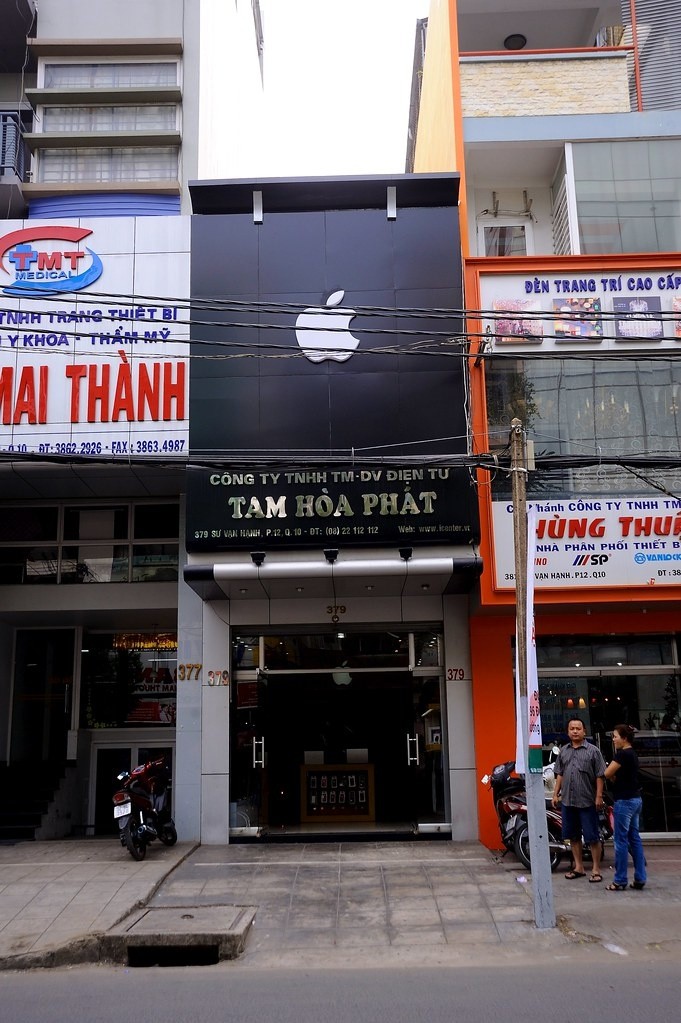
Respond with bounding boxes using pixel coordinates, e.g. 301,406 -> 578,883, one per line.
541,726 -> 681,831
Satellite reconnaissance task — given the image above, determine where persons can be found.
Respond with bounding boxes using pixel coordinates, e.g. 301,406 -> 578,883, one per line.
603,723 -> 647,891
160,702 -> 176,721
551,718 -> 607,883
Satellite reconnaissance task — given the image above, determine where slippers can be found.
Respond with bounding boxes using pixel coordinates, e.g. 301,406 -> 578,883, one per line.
589,872 -> 602,882
565,868 -> 587,879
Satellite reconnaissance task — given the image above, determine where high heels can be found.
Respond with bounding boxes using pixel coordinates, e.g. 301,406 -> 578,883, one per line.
630,880 -> 644,890
607,877 -> 627,891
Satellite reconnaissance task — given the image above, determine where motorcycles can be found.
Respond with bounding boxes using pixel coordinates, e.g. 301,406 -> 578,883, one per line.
481,745 -> 647,872
112,756 -> 178,861
